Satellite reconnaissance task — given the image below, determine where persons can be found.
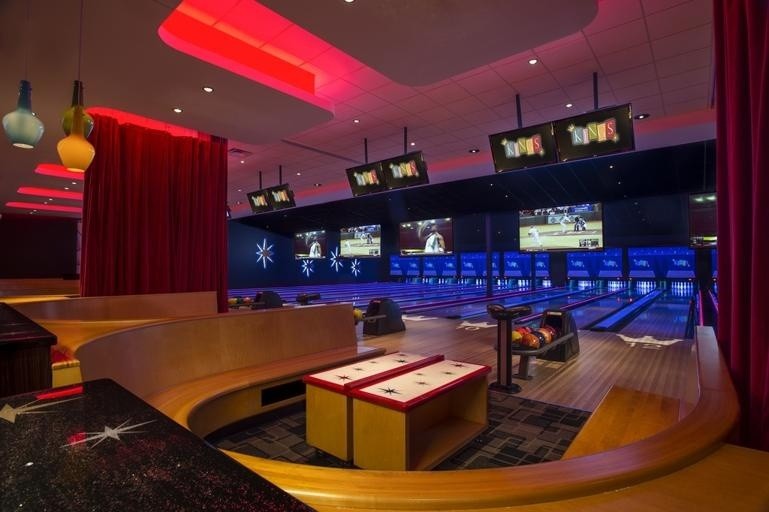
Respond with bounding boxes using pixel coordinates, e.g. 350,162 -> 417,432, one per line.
560,214 -> 571,233
529,226 -> 542,246
424,226 -> 445,253
309,237 -> 321,257
574,216 -> 586,231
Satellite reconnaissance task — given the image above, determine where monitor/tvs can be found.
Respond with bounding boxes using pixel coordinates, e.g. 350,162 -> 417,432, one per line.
345,160 -> 389,198
382,150 -> 430,191
551,103 -> 636,163
488,120 -> 560,173
266,184 -> 297,211
689,193 -> 718,250
247,188 -> 273,214
295,230 -> 326,260
340,225 -> 381,257
227,206 -> 231,217
517,204 -> 604,253
399,218 -> 457,256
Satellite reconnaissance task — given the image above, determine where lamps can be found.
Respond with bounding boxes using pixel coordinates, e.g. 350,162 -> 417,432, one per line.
1,2 -> 45,149
56,1 -> 95,172
56,0 -> 96,136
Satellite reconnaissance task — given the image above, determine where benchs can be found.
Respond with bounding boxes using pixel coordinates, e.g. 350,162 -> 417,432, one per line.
0,277 -> 80,301
7,291 -> 217,348
51,302 -> 387,438
217,325 -> 742,511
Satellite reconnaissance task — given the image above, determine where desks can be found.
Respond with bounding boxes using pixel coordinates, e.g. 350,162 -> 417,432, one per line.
303,350 -> 446,463
346,358 -> 491,471
0,301 -> 59,394
0,379 -> 317,512
